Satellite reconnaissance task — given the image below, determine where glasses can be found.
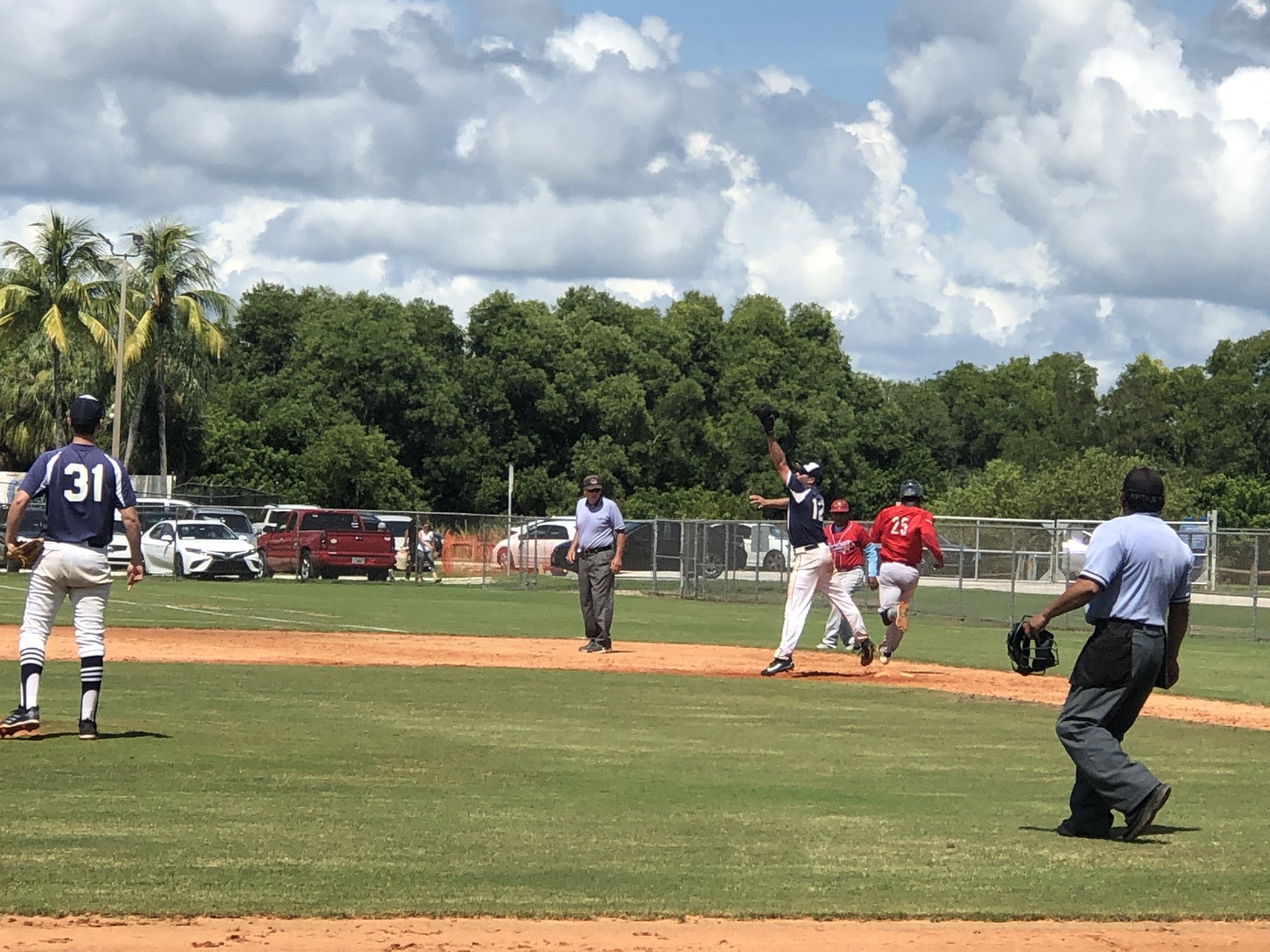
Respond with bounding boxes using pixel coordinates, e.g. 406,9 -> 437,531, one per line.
586,489 -> 600,493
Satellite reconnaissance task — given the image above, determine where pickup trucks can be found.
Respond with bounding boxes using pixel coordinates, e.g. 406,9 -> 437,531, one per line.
256,509 -> 397,582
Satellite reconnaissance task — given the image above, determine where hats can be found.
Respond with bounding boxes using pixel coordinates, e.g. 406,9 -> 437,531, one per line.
378,522 -> 385,529
583,475 -> 602,490
70,395 -> 106,420
1121,467 -> 1163,496
791,461 -> 823,486
829,500 -> 849,512
899,480 -> 922,500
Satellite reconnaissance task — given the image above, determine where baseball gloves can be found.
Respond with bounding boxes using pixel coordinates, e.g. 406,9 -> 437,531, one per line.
746,403 -> 780,434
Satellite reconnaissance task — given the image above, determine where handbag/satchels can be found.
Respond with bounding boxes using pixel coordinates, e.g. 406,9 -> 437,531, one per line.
396,549 -> 408,570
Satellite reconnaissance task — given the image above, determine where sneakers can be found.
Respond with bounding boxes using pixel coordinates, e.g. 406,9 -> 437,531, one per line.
858,639 -> 875,666
78,718 -> 97,739
579,639 -> 612,654
895,601 -> 909,634
0,705 -> 40,735
1122,783 -> 1171,842
1058,819 -> 1111,838
876,645 -> 891,664
761,658 -> 795,677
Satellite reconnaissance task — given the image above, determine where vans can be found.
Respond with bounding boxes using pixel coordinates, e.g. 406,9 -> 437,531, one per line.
252,503 -> 320,535
134,497 -> 195,524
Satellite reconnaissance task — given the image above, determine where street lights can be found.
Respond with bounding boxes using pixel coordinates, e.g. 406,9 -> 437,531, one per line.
97,231 -> 145,458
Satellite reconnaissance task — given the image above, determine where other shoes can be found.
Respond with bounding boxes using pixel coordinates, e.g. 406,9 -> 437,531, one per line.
434,578 -> 442,583
816,644 -> 831,649
845,645 -> 861,651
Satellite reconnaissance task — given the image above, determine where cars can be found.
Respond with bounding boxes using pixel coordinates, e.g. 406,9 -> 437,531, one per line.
186,507 -> 263,546
491,515 -> 581,576
140,520 -> 263,580
106,508 -> 143,565
0,502 -> 50,572
708,522 -> 796,572
415,525 -> 444,559
374,514 -> 430,570
551,522 -> 748,579
914,532 -> 981,579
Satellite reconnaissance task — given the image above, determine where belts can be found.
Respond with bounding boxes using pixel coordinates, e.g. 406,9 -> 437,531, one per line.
581,545 -> 612,554
805,542 -> 829,551
837,565 -> 863,572
882,560 -> 917,568
1098,623 -> 1162,632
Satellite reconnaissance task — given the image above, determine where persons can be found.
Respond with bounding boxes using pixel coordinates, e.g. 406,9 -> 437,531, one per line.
748,403 -> 877,676
567,475 -> 626,654
377,519 -> 442,582
1021,467 -> 1195,842
815,499 -> 878,651
0,393 -> 143,739
867,479 -> 946,666
